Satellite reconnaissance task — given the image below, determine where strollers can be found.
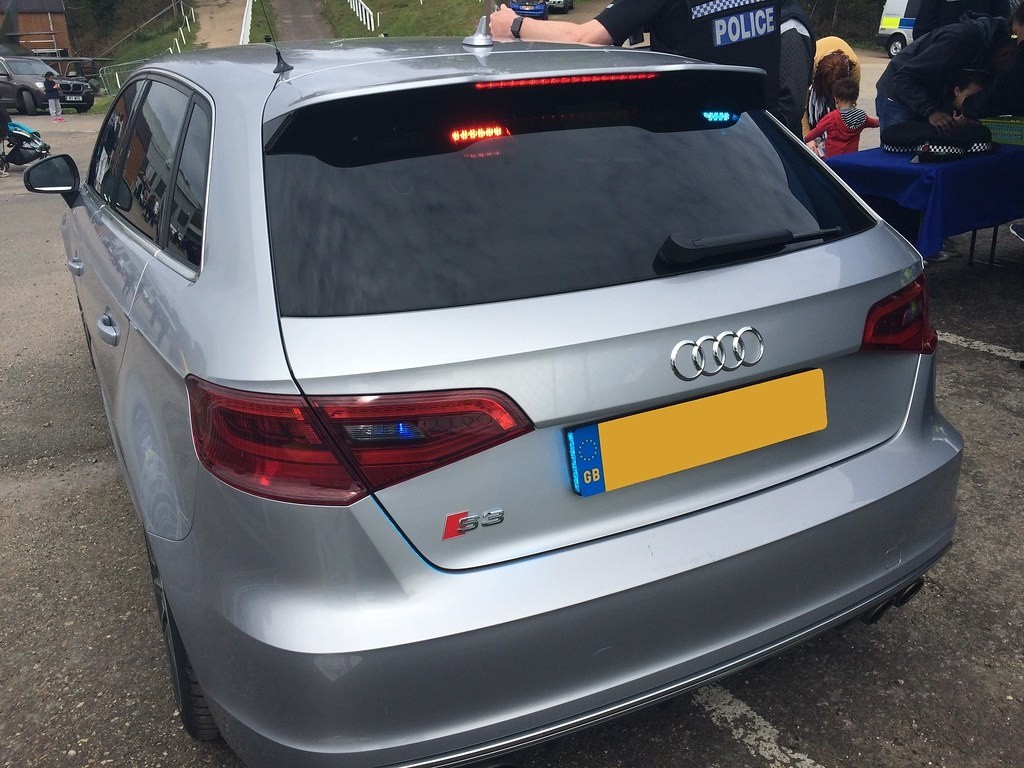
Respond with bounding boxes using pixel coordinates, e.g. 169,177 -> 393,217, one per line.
0,122 -> 51,173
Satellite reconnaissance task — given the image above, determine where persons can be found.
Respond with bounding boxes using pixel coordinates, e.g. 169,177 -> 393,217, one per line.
44,72 -> 66,122
96,124 -> 120,184
876,0 -> 1024,132
144,195 -> 159,228
489,0 -> 880,159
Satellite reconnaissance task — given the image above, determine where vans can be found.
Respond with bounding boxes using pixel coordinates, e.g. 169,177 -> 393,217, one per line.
875,0 -> 916,59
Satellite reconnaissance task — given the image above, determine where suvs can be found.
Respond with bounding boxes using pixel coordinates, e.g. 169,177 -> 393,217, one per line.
0,56 -> 94,116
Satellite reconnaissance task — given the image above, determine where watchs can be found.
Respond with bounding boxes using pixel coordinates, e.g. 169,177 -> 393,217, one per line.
512,17 -> 524,38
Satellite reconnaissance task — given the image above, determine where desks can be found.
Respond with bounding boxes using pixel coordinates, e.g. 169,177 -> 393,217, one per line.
822,142 -> 1024,269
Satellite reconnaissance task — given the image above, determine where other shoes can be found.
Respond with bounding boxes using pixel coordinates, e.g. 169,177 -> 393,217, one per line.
926,251 -> 949,261
52,117 -> 66,122
924,260 -> 928,267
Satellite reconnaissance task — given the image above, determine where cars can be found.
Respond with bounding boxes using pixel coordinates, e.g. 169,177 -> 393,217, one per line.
549,0 -> 574,14
508,0 -> 549,20
23,16 -> 965,768
